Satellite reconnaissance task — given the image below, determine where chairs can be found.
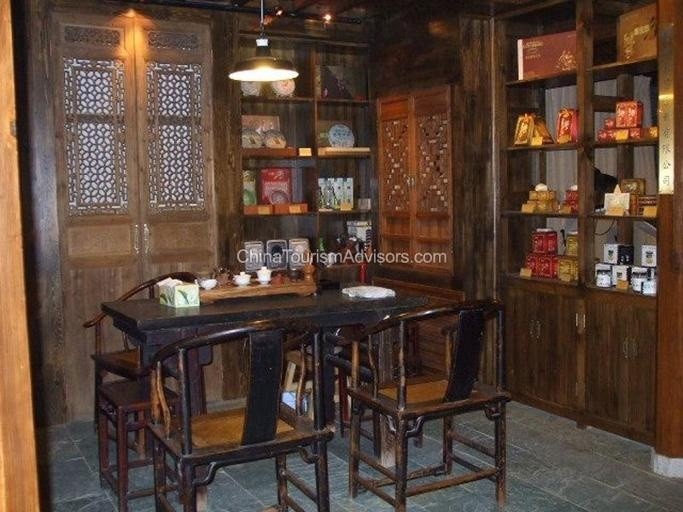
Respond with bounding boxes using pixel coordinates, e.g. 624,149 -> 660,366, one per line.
337,296 -> 510,511
281,322 -> 426,449
140,314 -> 338,511
83,270 -> 216,458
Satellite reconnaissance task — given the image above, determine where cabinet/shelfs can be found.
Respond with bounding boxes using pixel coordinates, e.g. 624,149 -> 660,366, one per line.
496,0 -> 659,449
206,9 -> 379,402
370,83 -> 490,377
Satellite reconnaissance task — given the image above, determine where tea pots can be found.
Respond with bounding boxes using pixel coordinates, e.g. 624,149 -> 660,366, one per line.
285,268 -> 305,283
211,266 -> 234,288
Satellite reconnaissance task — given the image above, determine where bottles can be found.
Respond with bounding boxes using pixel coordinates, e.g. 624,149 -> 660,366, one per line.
323,180 -> 334,209
606,250 -> 613,261
595,269 -> 612,289
632,271 -> 648,292
320,235 -> 327,267
645,251 -> 653,264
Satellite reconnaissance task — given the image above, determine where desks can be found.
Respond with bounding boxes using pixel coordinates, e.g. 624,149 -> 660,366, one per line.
96,286 -> 435,511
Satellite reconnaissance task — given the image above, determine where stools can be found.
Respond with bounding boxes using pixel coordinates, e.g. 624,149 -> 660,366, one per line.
95,377 -> 182,511
326,349 -> 423,451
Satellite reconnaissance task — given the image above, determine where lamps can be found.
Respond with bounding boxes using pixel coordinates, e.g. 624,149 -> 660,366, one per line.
225,1 -> 300,84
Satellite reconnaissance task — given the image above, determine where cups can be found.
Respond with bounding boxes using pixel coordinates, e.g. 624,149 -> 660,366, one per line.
274,274 -> 285,285
233,271 -> 252,284
200,278 -> 217,291
359,260 -> 367,283
255,266 -> 272,280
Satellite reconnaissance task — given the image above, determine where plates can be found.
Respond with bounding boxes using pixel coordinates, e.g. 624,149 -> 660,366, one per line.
232,281 -> 251,287
241,128 -> 262,149
270,79 -> 296,96
241,79 -> 261,95
255,278 -> 272,285
263,130 -> 287,149
328,123 -> 354,149
